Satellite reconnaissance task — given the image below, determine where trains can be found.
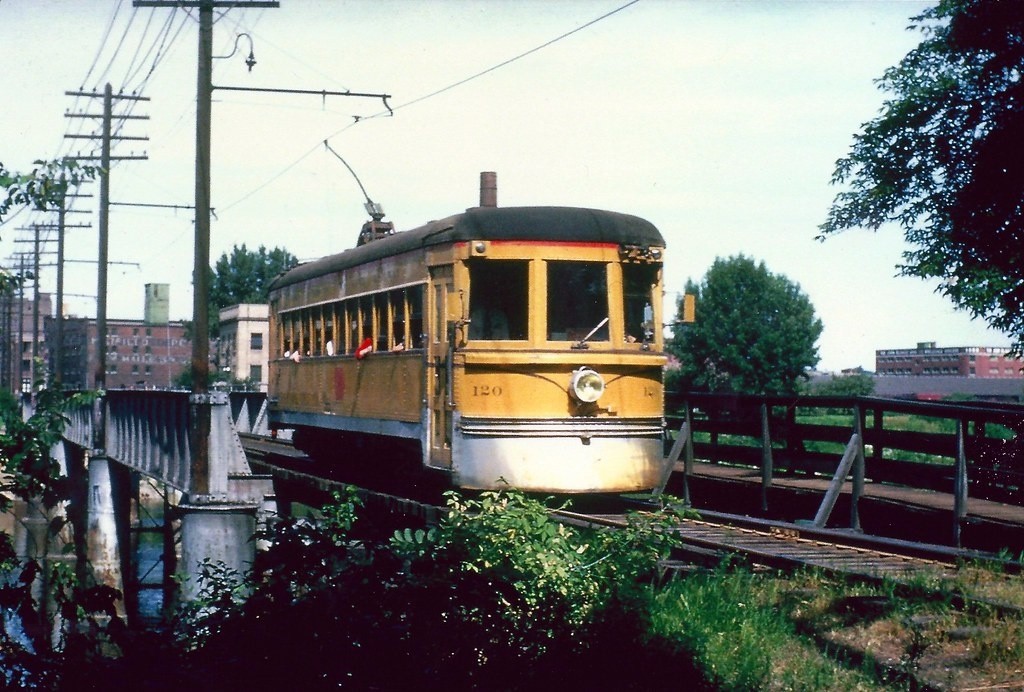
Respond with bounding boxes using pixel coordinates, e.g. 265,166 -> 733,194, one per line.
267,164 -> 696,495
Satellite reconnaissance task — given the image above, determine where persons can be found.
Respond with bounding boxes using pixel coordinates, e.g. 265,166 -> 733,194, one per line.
355,337 -> 373,359
327,340 -> 334,357
290,347 -> 300,363
393,340 -> 404,351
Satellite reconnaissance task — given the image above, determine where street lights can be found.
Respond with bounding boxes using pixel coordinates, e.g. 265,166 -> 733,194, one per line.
193,34 -> 256,446
